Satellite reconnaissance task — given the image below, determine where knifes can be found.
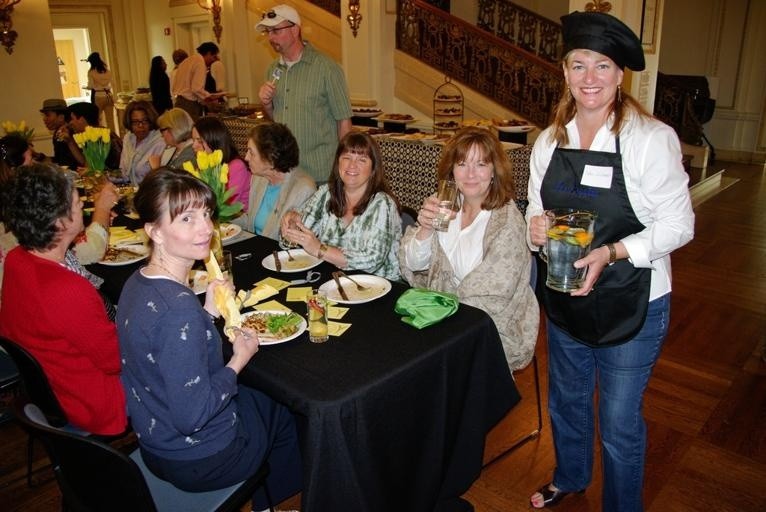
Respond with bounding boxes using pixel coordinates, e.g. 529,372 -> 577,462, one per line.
332,271 -> 349,300
273,247 -> 282,272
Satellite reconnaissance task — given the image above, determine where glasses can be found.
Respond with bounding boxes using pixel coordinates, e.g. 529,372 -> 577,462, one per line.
132,120 -> 150,124
260,24 -> 295,35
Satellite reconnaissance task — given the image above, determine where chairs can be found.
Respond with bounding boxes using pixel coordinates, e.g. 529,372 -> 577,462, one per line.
478,251 -> 544,471
0,336 -> 132,490
22,401 -> 274,511
397,204 -> 418,239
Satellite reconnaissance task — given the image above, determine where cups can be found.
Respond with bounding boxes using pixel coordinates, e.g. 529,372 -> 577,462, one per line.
431,178 -> 459,230
306,289 -> 329,344
279,206 -> 308,250
215,250 -> 233,271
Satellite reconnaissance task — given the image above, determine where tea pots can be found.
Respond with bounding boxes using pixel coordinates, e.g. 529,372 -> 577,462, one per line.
534,207 -> 598,292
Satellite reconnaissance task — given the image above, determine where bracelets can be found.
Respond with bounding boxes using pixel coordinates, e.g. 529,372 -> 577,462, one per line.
317,244 -> 328,259
602,243 -> 617,266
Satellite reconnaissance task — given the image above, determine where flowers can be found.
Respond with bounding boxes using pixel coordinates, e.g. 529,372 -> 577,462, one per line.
72,124 -> 112,177
1,118 -> 36,148
180,147 -> 245,223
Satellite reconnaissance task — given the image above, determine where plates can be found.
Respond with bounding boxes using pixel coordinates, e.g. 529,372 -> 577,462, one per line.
370,113 -> 420,124
223,310 -> 309,345
320,274 -> 391,305
351,108 -> 383,118
184,268 -> 212,297
262,248 -> 325,273
220,222 -> 241,242
492,119 -> 536,132
98,243 -> 150,266
390,132 -> 440,141
116,185 -> 137,196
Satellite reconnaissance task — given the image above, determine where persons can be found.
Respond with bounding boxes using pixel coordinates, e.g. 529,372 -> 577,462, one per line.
169,48 -> 188,99
524,8 -> 699,512
39,97 -> 316,241
173,41 -> 229,121
115,166 -> 308,512
280,131 -> 404,282
0,131 -> 118,266
81,51 -> 115,133
0,161 -> 133,439
396,125 -> 542,383
201,58 -> 228,118
150,55 -> 173,116
254,4 -> 354,188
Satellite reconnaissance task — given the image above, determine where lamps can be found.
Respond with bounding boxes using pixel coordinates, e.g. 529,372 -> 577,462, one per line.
0,0 -> 22,57
196,0 -> 224,45
585,1 -> 613,14
345,1 -> 363,40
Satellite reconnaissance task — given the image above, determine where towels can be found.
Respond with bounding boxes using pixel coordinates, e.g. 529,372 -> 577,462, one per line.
393,286 -> 461,331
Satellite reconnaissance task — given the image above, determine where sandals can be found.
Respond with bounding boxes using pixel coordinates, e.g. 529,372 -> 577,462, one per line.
530,482 -> 586,509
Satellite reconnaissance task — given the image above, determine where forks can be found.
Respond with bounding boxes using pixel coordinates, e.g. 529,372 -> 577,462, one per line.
338,270 -> 371,292
287,250 -> 296,263
236,289 -> 252,312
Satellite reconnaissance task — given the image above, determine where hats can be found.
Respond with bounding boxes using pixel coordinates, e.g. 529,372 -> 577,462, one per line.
560,10 -> 645,72
40,99 -> 67,113
254,4 -> 301,31
